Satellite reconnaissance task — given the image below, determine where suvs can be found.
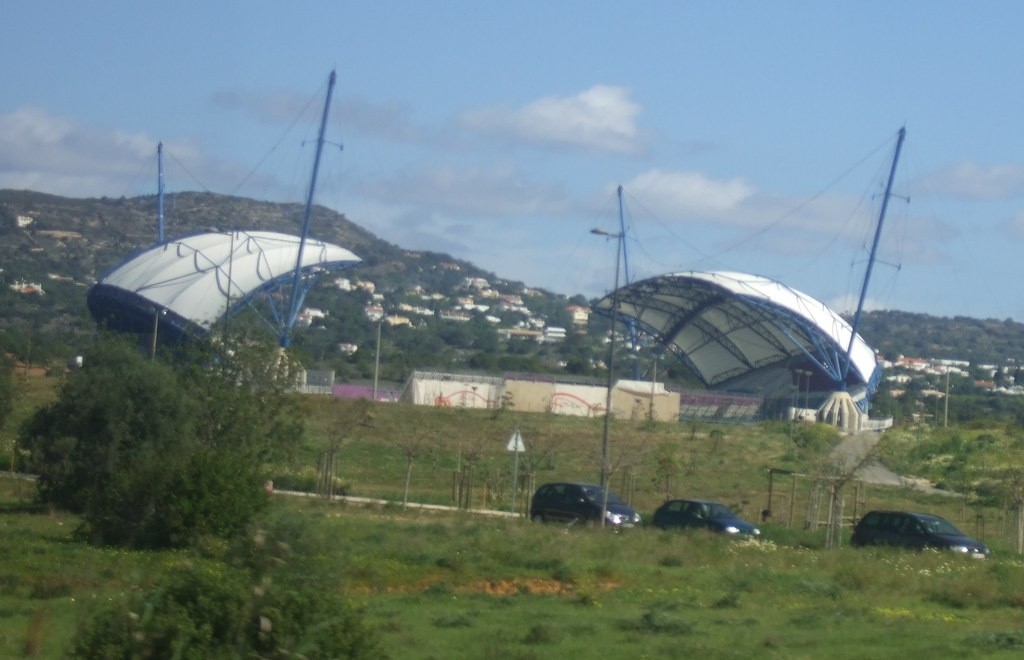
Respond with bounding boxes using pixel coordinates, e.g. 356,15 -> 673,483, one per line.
653,498 -> 761,540
530,482 -> 641,531
849,510 -> 991,559
